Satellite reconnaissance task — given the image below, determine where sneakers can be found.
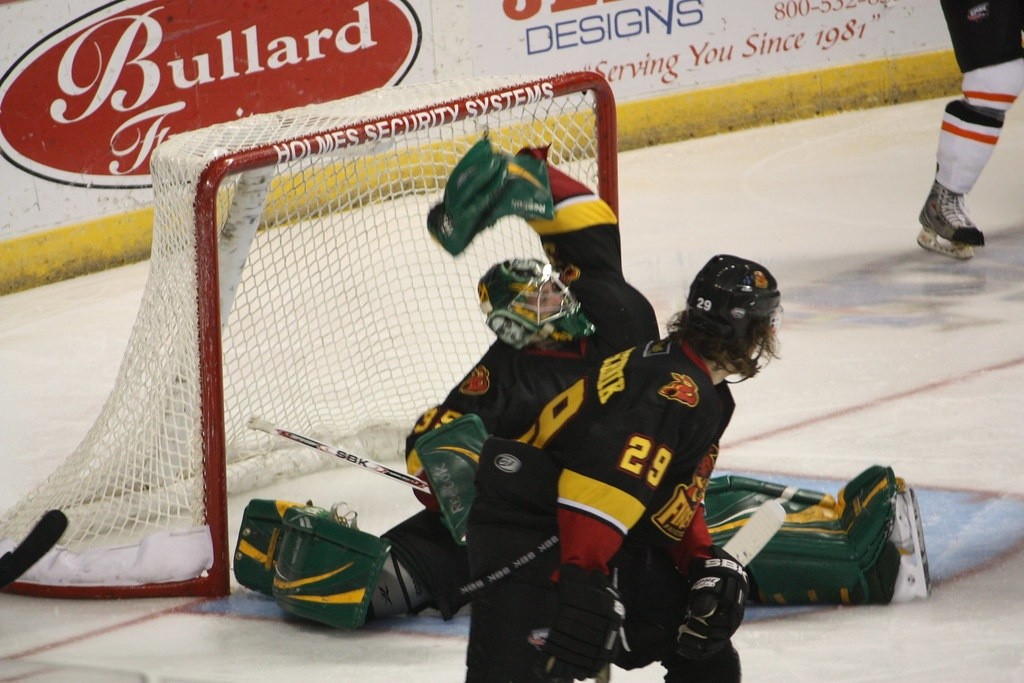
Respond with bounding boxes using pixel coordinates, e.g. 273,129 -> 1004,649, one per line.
917,181 -> 985,260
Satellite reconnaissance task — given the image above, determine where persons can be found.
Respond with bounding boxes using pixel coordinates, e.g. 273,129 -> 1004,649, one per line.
916,0 -> 1023,258
411,260 -> 593,505
465,252 -> 781,683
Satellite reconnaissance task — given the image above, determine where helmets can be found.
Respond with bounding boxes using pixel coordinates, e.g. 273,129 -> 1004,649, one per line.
686,254 -> 782,384
477,258 -> 594,350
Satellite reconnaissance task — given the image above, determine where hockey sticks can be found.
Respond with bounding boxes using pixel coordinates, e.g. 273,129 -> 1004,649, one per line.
245,418 -> 786,572
0,509 -> 68,589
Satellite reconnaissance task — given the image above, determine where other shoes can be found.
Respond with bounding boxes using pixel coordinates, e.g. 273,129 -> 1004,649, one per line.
887,483 -> 931,604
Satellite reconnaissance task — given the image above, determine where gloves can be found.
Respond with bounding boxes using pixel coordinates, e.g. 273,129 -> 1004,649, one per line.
674,547 -> 749,663
538,562 -> 617,681
